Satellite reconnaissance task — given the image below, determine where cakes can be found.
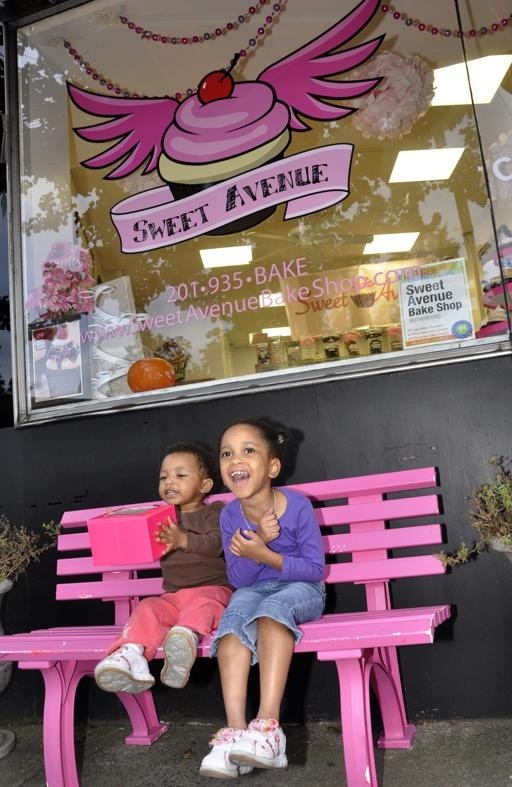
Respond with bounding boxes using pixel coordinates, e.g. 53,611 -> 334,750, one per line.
158,53 -> 291,236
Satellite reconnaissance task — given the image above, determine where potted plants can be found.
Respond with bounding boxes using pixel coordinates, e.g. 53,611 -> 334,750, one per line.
0,512 -> 63,763
435,453 -> 512,572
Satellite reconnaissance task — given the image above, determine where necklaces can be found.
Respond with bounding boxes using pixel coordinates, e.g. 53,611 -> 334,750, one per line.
240,488 -> 279,535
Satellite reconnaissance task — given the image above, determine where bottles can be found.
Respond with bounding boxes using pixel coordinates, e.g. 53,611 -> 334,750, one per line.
254,323 -> 405,369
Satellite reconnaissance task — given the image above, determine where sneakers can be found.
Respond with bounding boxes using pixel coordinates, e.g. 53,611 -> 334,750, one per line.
160,626 -> 199,689
198,726 -> 254,781
94,642 -> 156,694
228,718 -> 288,769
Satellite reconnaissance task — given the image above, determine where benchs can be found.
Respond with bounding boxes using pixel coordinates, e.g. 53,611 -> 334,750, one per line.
0,465 -> 452,787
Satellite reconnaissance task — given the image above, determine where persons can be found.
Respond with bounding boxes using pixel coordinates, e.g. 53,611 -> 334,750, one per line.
94,441 -> 237,696
198,415 -> 328,781
